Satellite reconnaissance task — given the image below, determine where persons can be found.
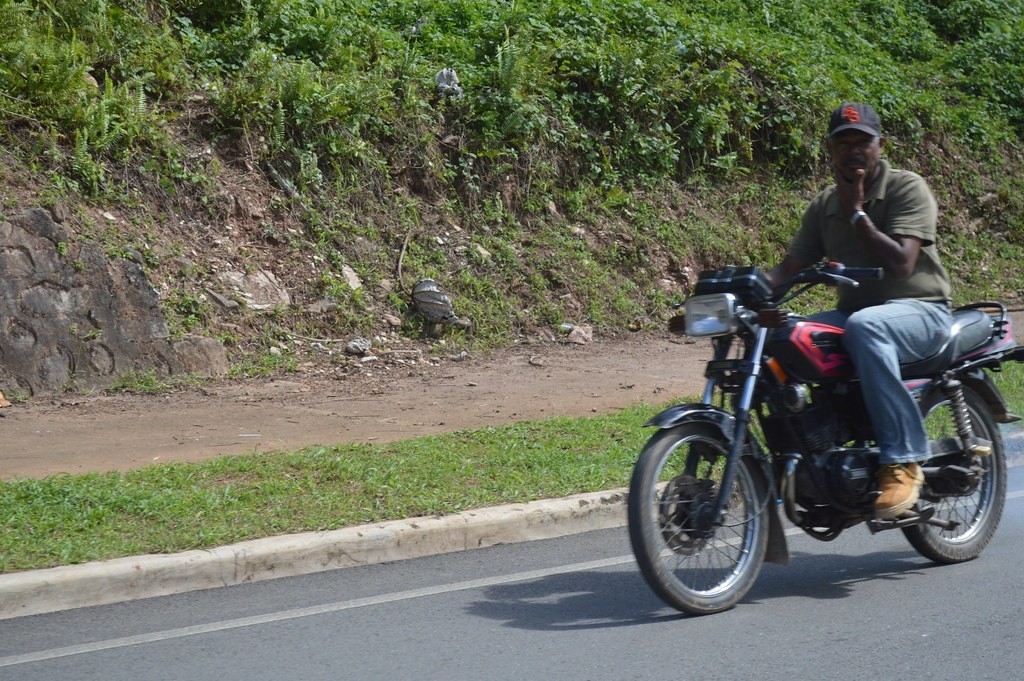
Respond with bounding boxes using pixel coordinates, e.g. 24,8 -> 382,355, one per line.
768,103 -> 953,519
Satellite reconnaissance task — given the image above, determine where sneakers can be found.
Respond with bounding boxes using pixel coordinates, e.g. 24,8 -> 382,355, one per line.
874,462 -> 925,519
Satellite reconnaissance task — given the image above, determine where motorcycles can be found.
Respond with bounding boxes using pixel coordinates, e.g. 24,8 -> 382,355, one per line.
625,257 -> 1024,616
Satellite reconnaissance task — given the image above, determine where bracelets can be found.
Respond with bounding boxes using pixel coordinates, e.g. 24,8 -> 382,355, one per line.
851,211 -> 867,225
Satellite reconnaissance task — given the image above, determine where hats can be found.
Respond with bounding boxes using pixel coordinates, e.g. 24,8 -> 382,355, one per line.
828,102 -> 881,138
